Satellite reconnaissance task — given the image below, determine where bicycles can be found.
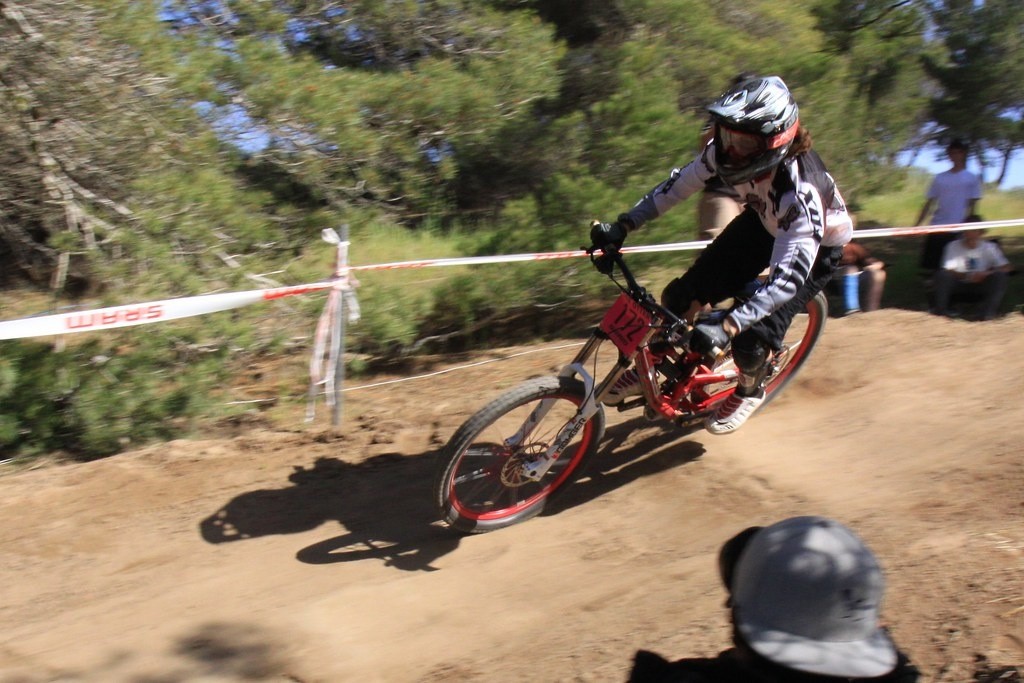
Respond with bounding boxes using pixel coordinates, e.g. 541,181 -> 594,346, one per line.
431,218 -> 829,533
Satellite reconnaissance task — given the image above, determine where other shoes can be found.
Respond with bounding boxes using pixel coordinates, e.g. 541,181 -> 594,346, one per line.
934,300 -> 953,315
981,305 -> 997,320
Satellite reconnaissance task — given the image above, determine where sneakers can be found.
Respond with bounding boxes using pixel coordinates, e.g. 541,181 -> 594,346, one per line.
705,389 -> 766,433
596,368 -> 644,407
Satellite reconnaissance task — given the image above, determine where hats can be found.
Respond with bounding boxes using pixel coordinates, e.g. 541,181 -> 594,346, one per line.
727,516 -> 897,677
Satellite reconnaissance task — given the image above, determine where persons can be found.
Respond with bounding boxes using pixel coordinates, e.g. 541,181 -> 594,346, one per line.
595,73 -> 853,437
730,510 -> 923,682
697,70 -> 762,269
934,214 -> 1022,320
822,201 -> 885,310
907,141 -> 984,309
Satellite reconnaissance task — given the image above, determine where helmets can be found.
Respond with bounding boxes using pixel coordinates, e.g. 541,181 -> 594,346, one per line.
706,76 -> 800,184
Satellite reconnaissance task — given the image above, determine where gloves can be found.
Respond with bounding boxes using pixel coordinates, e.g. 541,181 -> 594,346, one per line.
689,322 -> 731,355
590,222 -> 628,258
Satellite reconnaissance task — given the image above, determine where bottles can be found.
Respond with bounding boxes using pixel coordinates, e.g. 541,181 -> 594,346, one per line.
967,255 -> 979,282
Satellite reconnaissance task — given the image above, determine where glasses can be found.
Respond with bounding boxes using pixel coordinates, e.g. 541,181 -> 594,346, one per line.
719,128 -> 759,155
